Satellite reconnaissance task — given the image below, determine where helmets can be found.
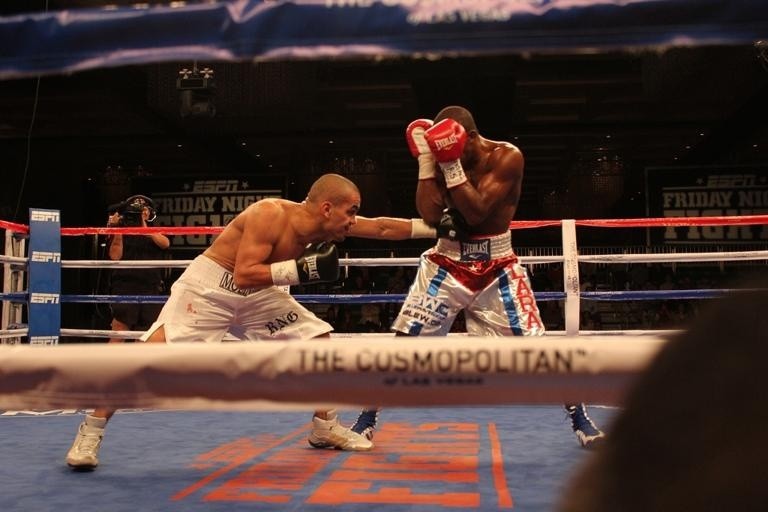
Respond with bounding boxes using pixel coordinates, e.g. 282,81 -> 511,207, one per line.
123,194 -> 158,225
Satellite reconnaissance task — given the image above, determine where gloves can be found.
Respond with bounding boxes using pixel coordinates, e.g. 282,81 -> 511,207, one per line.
410,206 -> 467,241
423,117 -> 468,191
405,118 -> 440,180
270,240 -> 341,286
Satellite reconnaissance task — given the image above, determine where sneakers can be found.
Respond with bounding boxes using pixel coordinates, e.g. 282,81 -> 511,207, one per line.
566,401 -> 609,450
307,409 -> 375,450
65,413 -> 107,469
349,410 -> 380,441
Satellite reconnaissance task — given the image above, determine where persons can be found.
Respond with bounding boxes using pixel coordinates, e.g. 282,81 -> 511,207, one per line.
325,266 -> 415,333
106,195 -> 169,343
352,105 -> 607,452
66,173 -> 437,468
531,263 -> 603,330
